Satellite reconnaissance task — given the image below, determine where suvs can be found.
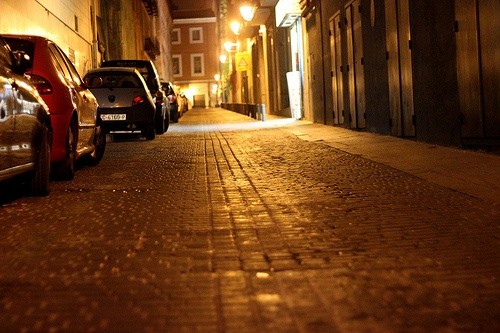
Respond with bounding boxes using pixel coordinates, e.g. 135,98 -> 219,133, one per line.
100,59 -> 170,135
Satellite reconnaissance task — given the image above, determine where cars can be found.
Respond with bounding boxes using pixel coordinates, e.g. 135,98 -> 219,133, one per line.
0,34 -> 106,178
0,38 -> 52,201
160,82 -> 189,123
82,66 -> 155,140
